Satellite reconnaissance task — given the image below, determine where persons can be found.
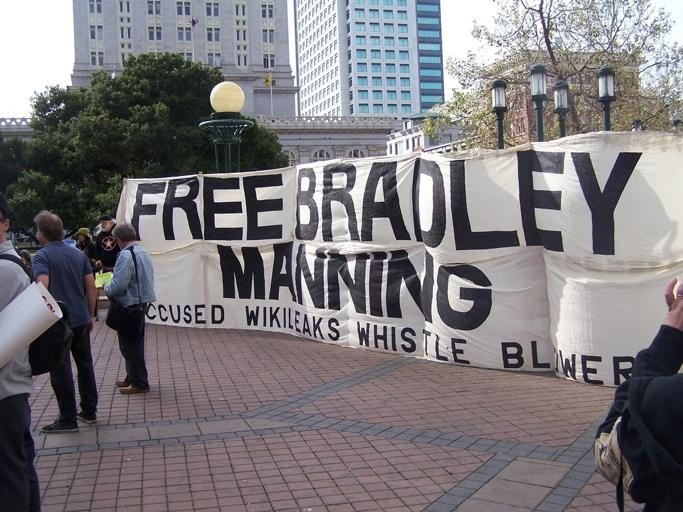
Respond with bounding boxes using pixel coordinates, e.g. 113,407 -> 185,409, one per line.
595,278 -> 683,512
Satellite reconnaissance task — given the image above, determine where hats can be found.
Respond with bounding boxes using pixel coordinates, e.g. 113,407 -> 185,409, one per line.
74,228 -> 92,239
99,215 -> 114,221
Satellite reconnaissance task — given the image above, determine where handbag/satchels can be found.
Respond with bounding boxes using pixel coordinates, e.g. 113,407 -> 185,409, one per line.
104,300 -> 144,338
0,254 -> 73,376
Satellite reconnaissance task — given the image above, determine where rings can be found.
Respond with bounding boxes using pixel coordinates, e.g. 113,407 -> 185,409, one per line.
677,295 -> 683,297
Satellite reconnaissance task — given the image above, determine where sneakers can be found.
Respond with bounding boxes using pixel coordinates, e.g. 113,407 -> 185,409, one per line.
116,380 -> 149,394
41,413 -> 98,432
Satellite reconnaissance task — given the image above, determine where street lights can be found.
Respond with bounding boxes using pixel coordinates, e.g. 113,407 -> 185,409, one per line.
199,79 -> 256,172
486,63 -> 683,150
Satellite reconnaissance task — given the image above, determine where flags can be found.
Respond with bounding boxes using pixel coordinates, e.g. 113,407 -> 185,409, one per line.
262,74 -> 271,86
